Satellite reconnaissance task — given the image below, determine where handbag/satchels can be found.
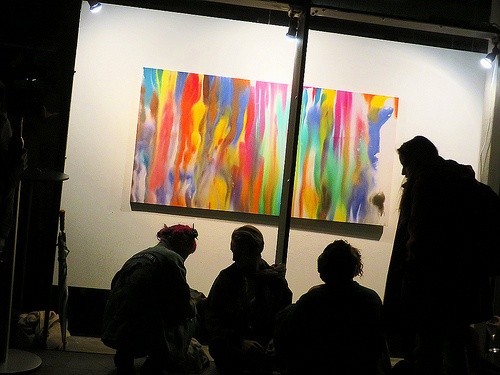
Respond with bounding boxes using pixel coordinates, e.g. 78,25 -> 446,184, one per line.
18,310 -> 65,350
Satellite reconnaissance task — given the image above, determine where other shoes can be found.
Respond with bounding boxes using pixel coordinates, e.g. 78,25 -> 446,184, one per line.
114,355 -> 136,375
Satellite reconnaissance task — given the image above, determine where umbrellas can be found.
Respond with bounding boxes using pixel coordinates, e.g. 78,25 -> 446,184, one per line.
58,210 -> 70,352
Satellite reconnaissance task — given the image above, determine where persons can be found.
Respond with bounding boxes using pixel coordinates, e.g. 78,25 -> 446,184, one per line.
383,136 -> 499,375
196,224 -> 294,375
271,239 -> 392,375
99,224 -> 200,374
